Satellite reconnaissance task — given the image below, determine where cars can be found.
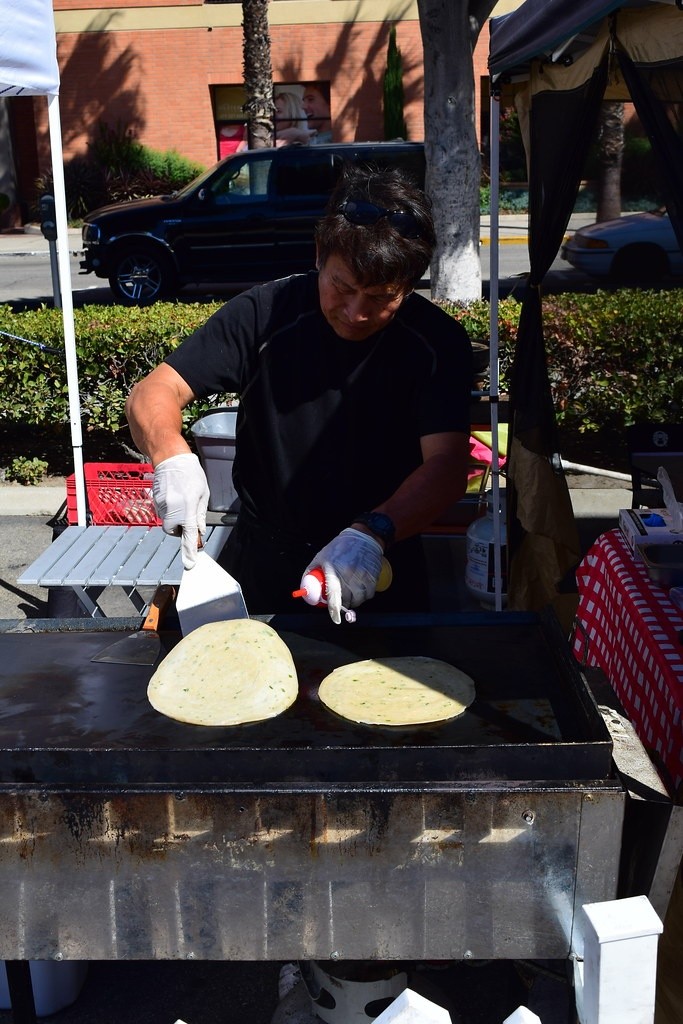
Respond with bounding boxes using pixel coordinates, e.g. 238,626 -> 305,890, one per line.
560,200 -> 683,288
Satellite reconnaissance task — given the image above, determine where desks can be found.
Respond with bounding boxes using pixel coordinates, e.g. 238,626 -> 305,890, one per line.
16,525 -> 231,618
570,528 -> 682,925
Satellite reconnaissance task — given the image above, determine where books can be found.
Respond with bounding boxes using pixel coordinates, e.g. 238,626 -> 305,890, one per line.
619,508 -> 683,561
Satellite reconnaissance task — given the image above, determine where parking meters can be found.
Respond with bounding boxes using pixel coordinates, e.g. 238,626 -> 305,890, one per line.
37,192 -> 63,309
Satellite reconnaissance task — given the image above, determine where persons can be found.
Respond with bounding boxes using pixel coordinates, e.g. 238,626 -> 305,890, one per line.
126,169 -> 469,1000
275,83 -> 330,144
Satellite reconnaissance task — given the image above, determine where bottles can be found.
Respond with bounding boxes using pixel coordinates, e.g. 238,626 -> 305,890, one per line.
467,489 -> 510,600
293,556 -> 392,608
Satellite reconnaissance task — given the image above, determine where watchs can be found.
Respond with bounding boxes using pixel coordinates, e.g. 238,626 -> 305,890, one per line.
353,512 -> 394,542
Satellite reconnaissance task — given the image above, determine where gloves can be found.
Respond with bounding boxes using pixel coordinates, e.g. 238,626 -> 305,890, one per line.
152,453 -> 209,569
303,527 -> 383,625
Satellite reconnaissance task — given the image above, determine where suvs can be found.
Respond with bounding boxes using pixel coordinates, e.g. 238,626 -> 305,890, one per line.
80,141 -> 424,311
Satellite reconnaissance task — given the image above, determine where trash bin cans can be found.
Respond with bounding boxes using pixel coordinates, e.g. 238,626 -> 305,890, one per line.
188,405 -> 242,513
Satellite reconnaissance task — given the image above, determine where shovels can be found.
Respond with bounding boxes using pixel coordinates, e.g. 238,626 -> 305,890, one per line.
174,525 -> 251,639
89,584 -> 176,666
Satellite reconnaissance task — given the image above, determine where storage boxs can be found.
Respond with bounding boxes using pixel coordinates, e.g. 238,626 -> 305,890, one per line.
66,463 -> 164,526
